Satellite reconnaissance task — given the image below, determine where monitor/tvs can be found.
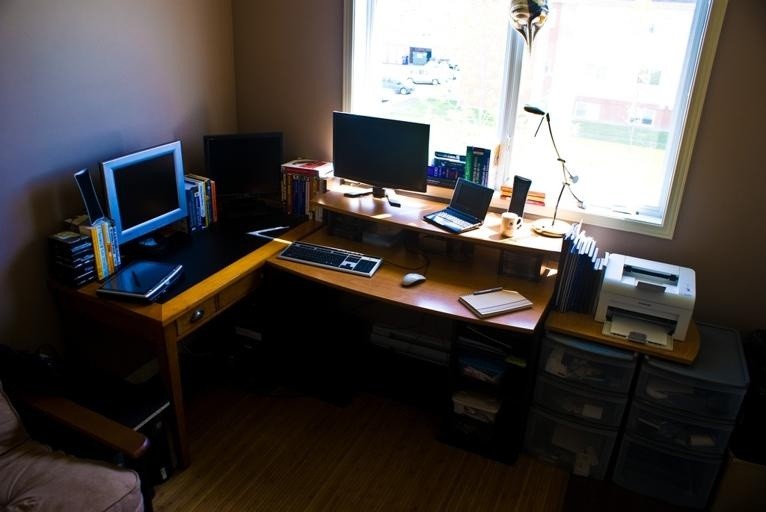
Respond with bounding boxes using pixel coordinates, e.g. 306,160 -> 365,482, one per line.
100,139 -> 188,245
333,110 -> 430,206
203,132 -> 282,195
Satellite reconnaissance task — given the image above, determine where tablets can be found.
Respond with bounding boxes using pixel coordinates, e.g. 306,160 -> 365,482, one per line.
94,261 -> 184,301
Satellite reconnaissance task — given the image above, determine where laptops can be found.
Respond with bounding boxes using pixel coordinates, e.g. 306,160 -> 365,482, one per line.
422,176 -> 494,234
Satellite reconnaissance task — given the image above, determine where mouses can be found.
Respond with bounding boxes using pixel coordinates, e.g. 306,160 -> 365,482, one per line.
401,273 -> 426,287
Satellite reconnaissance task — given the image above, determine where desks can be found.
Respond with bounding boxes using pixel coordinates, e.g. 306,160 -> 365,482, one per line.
50,204 -> 329,467
258,187 -> 568,463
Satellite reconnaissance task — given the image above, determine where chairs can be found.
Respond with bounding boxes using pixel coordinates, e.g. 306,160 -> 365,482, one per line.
0,360 -> 170,511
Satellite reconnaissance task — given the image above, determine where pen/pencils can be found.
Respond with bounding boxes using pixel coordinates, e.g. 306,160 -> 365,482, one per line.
473,287 -> 504,295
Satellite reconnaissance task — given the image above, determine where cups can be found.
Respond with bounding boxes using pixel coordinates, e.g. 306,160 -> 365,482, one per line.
501,211 -> 523,238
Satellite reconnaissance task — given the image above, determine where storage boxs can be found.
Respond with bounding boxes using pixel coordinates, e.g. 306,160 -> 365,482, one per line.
709,441 -> 766,510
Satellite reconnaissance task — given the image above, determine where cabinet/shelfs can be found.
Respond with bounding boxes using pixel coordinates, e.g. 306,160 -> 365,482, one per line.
609,315 -> 752,504
527,295 -> 637,481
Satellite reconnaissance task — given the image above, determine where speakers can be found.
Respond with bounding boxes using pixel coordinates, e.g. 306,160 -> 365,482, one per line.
75,169 -> 101,225
510,176 -> 531,218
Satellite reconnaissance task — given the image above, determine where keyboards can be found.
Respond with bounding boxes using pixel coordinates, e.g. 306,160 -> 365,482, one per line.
277,241 -> 383,278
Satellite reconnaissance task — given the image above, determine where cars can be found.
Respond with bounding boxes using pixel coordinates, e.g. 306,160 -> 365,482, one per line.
380,51 -> 460,104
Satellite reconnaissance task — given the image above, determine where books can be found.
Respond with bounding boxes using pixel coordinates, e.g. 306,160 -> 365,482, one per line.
501,179 -> 546,207
281,160 -> 337,223
184,172 -> 218,234
52,215 -> 120,289
458,289 -> 534,318
558,225 -> 603,315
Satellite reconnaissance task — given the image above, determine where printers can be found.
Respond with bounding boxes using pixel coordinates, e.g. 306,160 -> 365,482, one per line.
590,252 -> 695,352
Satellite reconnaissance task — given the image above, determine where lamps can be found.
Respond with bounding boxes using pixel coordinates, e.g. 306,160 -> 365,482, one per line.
526,102 -> 587,239
